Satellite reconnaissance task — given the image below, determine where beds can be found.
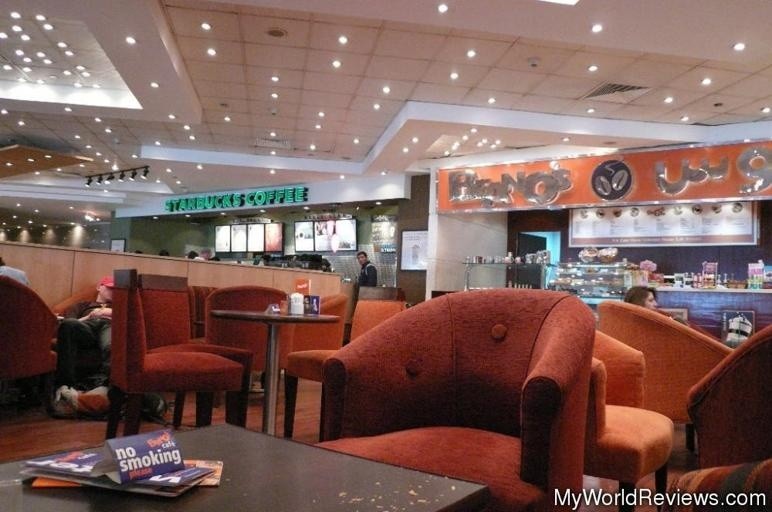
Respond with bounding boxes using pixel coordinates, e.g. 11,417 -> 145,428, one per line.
0,423 -> 489,512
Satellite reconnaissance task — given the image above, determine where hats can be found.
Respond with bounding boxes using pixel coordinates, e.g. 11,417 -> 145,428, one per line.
99,275 -> 114,287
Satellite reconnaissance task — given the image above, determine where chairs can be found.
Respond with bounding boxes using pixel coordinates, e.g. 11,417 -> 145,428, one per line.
0,274 -> 62,420
51,282 -> 113,320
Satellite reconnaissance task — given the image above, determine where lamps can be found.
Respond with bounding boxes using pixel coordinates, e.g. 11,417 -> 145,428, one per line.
84,165 -> 150,186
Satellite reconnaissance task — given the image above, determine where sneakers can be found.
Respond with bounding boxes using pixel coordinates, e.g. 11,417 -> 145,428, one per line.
145,393 -> 167,417
69,381 -> 95,392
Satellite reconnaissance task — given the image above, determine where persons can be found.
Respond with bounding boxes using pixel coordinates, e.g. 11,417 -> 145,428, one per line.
623,285 -> 659,312
0,257 -> 31,286
159,249 -> 169,256
357,250 -> 378,288
187,250 -> 205,260
52,276 -> 115,417
